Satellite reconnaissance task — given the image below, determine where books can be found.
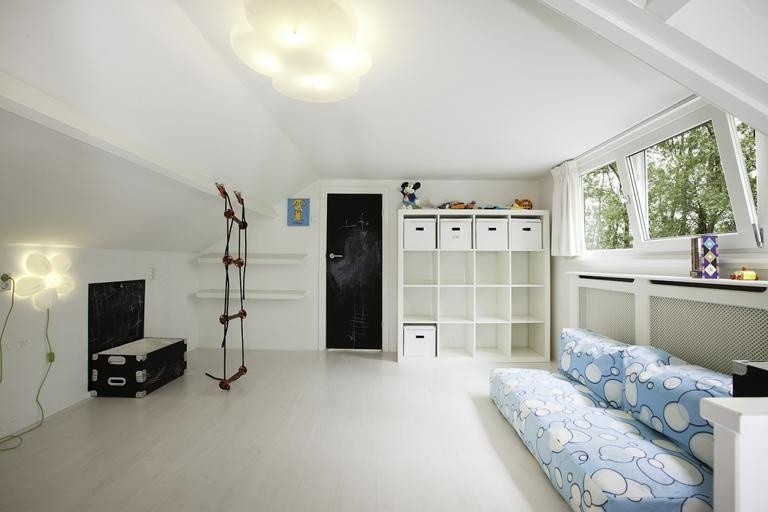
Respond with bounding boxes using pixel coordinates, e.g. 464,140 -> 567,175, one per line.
689,236 -> 718,278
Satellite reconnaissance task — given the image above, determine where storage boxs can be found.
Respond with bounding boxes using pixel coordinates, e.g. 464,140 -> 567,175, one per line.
88,335 -> 188,400
404,216 -> 544,253
402,325 -> 437,358
730,357 -> 767,397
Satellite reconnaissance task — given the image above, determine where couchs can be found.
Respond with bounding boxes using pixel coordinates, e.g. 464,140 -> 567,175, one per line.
489,326 -> 734,512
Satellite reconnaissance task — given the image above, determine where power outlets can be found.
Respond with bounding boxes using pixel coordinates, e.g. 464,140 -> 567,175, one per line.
0,272 -> 12,293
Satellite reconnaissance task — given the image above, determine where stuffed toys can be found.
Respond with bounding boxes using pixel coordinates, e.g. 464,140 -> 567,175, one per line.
400,181 -> 420,208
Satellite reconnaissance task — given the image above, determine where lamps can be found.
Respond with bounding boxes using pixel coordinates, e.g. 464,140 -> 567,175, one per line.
12,250 -> 77,310
228,0 -> 404,105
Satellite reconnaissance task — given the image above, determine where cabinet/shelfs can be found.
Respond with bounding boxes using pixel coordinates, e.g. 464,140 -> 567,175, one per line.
398,209 -> 552,364
197,251 -> 307,301
